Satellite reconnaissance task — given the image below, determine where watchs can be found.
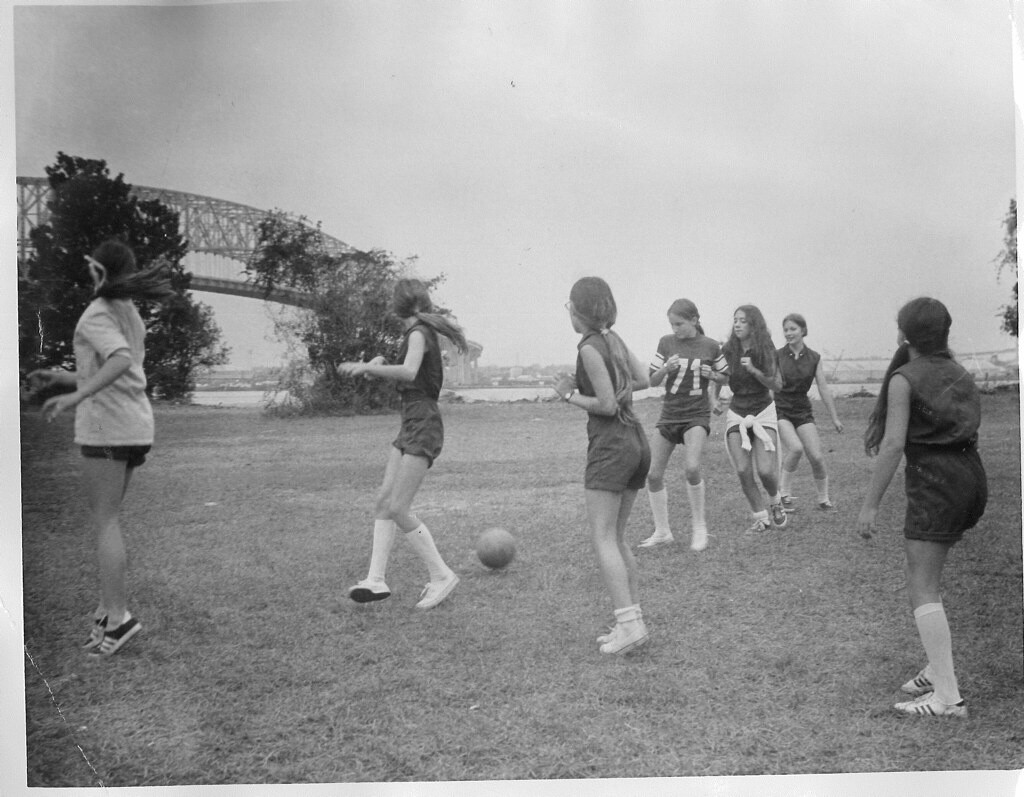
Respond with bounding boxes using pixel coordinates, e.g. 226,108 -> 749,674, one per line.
565,390 -> 574,402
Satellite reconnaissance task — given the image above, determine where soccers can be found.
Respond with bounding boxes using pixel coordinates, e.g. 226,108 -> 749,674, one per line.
478,528 -> 516,568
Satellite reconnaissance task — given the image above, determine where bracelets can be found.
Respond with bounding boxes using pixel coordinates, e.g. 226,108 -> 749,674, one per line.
664,363 -> 670,373
712,372 -> 717,380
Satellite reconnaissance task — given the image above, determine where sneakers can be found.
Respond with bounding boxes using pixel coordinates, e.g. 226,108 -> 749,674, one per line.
416,572 -> 459,609
900,667 -> 934,694
640,532 -> 673,548
600,625 -> 648,656
780,495 -> 795,512
690,529 -> 710,550
745,519 -> 769,536
82,616 -> 107,648
818,502 -> 837,514
348,578 -> 391,602
769,496 -> 787,527
895,692 -> 967,720
598,629 -> 616,644
88,611 -> 141,657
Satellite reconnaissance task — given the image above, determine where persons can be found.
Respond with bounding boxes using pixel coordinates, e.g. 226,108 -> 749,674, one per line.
775,314 -> 844,512
712,304 -> 787,533
27,242 -> 174,657
638,298 -> 732,551
855,297 -> 987,718
552,276 -> 652,653
337,278 -> 469,609
